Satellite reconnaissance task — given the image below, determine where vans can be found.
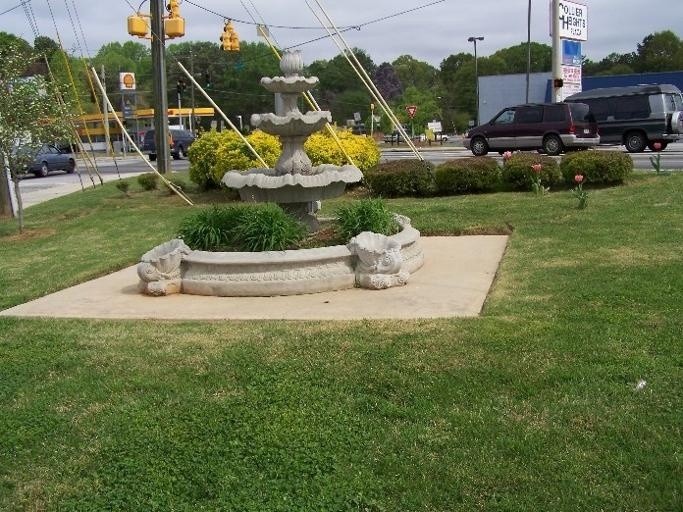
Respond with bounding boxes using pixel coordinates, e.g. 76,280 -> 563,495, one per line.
384,128 -> 411,142
547,83 -> 682,150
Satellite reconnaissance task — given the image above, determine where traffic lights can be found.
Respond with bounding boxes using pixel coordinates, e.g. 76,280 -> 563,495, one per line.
204,71 -> 214,89
219,33 -> 225,51
553,77 -> 565,87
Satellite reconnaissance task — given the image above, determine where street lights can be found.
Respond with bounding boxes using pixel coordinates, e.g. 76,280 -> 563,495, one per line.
466,36 -> 484,126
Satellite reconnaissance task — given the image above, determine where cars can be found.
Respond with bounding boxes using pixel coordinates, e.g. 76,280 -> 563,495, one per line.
414,132 -> 448,141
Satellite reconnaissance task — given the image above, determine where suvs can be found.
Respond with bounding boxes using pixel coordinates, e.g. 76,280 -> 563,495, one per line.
11,143 -> 76,176
141,127 -> 195,160
463,101 -> 601,156
